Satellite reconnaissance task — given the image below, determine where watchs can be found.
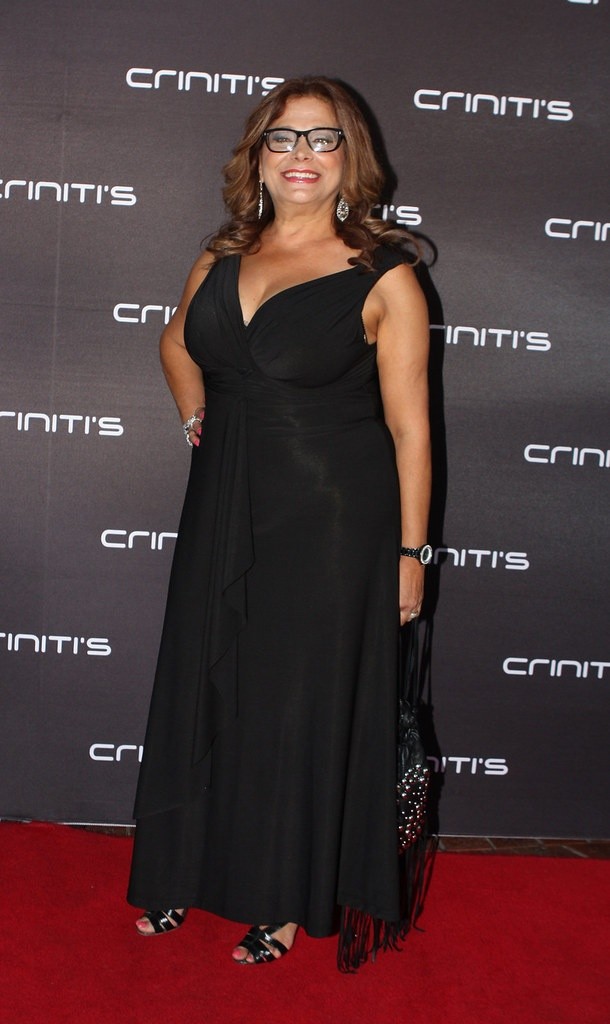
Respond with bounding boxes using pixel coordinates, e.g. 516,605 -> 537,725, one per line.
401,544 -> 432,565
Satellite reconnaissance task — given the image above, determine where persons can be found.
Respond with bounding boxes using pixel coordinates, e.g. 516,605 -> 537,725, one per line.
125,73 -> 440,973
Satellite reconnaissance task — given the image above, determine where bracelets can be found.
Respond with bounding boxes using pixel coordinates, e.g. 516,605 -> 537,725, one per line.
182,416 -> 202,447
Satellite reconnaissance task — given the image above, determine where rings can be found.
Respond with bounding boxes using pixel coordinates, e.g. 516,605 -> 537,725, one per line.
190,416 -> 201,430
410,612 -> 418,618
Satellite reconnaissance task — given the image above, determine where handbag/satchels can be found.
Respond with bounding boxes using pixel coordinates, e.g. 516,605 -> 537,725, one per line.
336,616 -> 433,972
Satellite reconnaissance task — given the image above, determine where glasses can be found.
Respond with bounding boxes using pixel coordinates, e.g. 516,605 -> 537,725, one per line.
262,127 -> 346,153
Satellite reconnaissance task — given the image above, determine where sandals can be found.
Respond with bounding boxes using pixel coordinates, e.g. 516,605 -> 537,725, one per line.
232,920 -> 293,966
135,908 -> 186,936
